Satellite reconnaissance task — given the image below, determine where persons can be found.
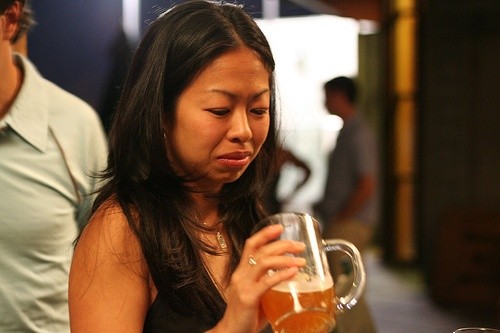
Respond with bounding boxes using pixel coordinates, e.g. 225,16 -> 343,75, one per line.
0,1 -> 110,333
312,75 -> 378,333
64,0 -> 307,333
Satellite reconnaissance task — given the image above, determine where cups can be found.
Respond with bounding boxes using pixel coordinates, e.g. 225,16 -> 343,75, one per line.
250,213 -> 366,333
452,327 -> 500,333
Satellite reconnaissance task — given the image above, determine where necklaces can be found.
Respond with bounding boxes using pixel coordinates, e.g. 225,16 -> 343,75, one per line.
185,207 -> 227,249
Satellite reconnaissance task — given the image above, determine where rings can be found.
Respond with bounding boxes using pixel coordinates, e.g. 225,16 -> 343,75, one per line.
248,258 -> 256,265
